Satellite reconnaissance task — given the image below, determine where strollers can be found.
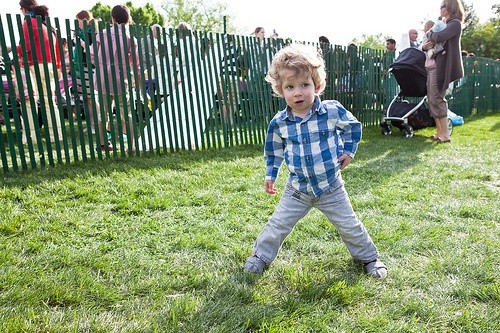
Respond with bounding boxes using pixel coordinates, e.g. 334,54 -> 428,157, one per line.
379,48 -> 436,138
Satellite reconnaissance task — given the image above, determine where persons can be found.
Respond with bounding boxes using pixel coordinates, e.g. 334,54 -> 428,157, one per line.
0,1 -> 500,152
243,44 -> 389,280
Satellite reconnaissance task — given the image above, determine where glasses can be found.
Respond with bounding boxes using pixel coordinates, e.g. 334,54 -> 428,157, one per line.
441,3 -> 447,8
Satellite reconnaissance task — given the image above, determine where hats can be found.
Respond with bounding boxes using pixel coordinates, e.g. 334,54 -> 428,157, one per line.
432,20 -> 448,33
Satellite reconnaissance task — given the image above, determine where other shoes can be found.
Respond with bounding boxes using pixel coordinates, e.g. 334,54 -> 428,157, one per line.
434,137 -> 451,144
107,132 -> 111,140
96,144 -> 118,154
365,258 -> 388,280
244,256 -> 268,276
429,134 -> 440,141
122,133 -> 128,142
